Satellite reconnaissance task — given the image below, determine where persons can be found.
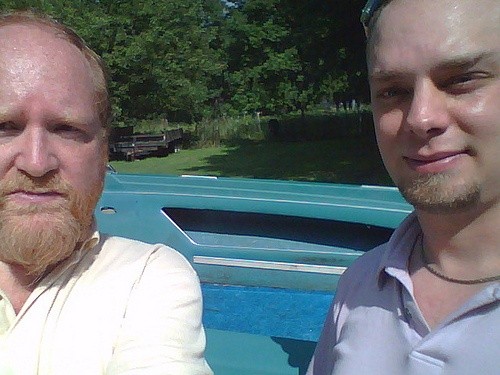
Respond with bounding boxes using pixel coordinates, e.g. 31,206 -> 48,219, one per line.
304,0 -> 500,375
0,12 -> 214,375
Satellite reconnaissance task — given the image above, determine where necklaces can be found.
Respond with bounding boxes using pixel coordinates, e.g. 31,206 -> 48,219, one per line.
420,233 -> 499,284
